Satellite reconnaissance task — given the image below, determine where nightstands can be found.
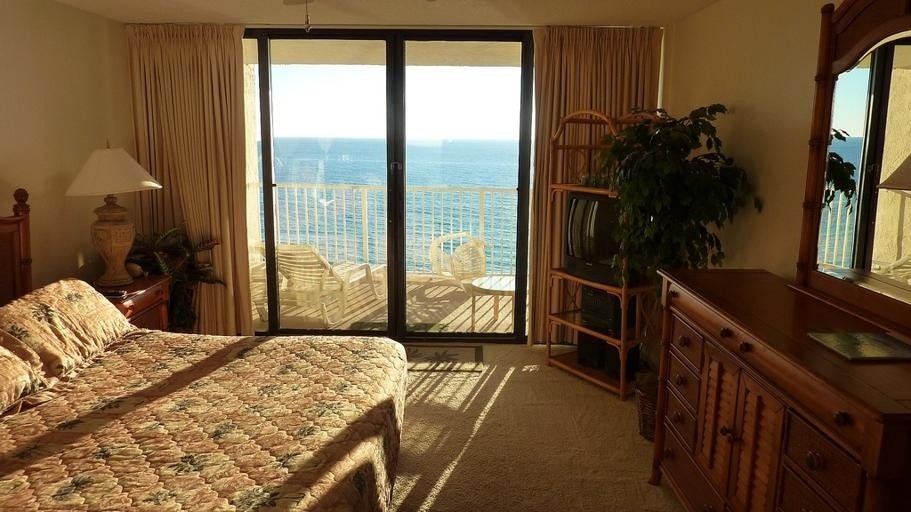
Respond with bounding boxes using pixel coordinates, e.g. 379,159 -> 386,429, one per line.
92,274 -> 172,332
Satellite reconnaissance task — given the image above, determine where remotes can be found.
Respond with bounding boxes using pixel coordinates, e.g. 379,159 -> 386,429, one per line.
103,289 -> 125,296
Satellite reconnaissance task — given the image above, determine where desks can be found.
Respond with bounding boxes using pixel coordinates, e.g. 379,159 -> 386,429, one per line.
471,276 -> 516,330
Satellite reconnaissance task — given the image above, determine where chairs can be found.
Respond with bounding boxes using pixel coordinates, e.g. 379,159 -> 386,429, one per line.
253,240 -> 387,330
429,232 -> 495,295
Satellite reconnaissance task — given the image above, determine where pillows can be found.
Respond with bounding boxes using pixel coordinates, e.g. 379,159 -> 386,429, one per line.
0,328 -> 70,425
0,278 -> 162,388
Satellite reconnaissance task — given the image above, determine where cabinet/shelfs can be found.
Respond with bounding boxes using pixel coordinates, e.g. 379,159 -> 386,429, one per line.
648,268 -> 910,510
546,110 -> 666,401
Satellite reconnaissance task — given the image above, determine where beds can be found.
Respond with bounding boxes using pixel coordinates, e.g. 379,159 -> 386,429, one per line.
0,188 -> 408,512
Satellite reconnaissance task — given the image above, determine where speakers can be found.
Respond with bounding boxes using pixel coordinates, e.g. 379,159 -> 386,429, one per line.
578,285 -> 640,381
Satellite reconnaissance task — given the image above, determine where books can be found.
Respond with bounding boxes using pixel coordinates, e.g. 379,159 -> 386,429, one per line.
810,326 -> 910,363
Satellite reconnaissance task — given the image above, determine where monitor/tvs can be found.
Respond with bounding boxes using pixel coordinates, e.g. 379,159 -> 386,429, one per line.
564,191 -> 641,289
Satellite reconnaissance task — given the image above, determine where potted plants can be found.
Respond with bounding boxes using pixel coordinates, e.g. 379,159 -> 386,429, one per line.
593,103 -> 748,443
128,220 -> 227,333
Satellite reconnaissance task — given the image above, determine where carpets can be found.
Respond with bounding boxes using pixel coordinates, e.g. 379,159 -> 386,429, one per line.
403,345 -> 484,371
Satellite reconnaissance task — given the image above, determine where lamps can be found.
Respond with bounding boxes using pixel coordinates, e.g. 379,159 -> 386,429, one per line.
65,137 -> 162,288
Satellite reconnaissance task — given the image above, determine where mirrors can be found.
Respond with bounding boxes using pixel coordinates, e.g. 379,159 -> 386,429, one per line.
787,0 -> 910,342
